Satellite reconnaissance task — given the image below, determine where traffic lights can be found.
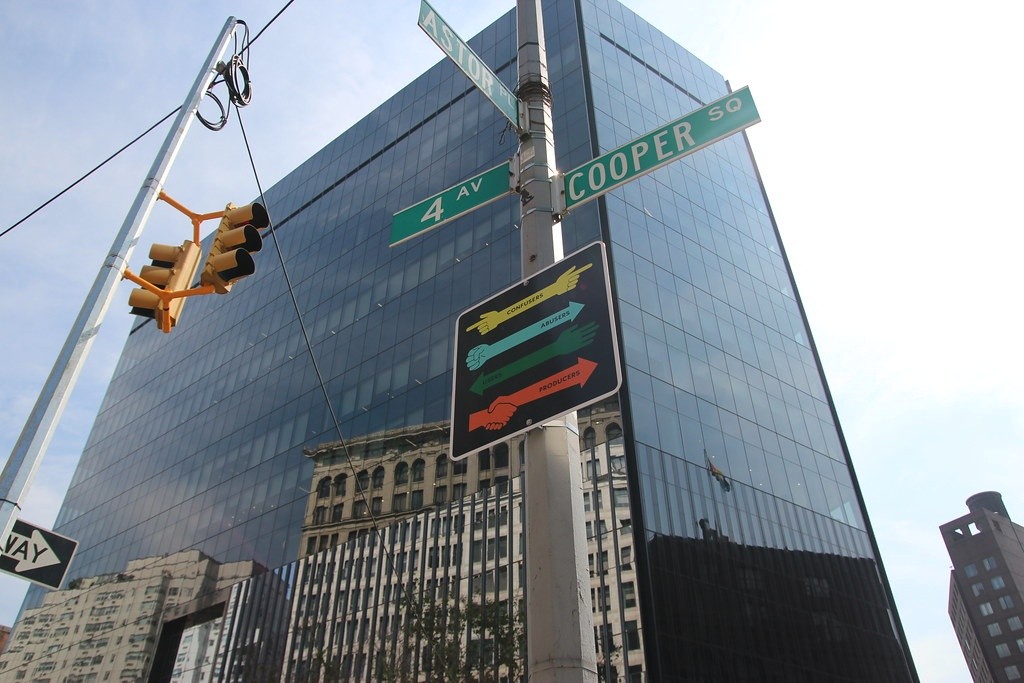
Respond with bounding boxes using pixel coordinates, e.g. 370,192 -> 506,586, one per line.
128,238 -> 201,330
200,199 -> 270,294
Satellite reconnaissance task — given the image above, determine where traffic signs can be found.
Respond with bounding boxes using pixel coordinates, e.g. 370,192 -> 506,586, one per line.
387,158 -> 513,248
417,0 -> 520,133
0,519 -> 80,592
565,87 -> 762,211
448,242 -> 623,463
